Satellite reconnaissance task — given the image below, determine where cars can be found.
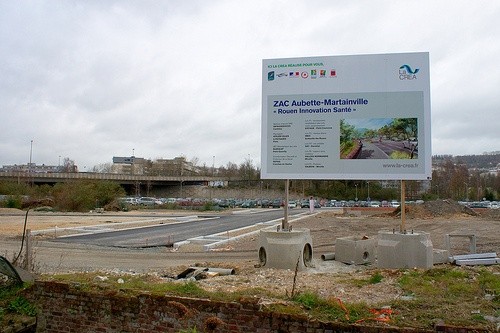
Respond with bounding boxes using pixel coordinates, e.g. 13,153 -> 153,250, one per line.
114,192 -> 500,210
